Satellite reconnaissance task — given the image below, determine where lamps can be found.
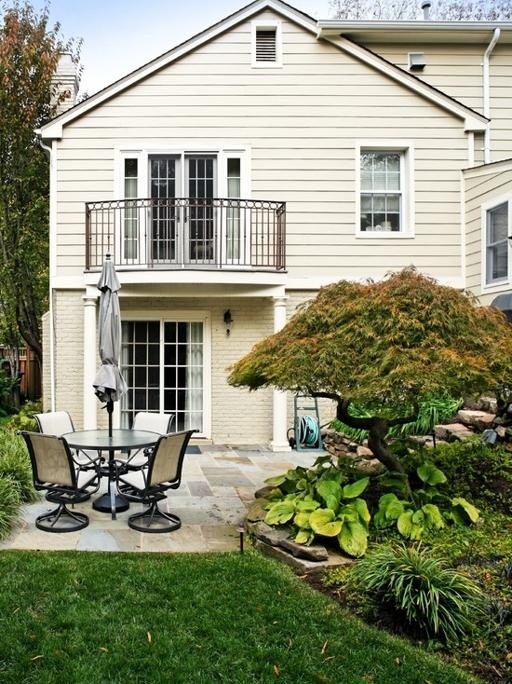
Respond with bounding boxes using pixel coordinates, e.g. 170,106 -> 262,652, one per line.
224,309 -> 233,335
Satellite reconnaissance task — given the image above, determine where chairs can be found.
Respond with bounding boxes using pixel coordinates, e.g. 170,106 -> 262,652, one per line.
33,412 -> 89,503
117,412 -> 175,501
110,428 -> 199,533
17,431 -> 105,532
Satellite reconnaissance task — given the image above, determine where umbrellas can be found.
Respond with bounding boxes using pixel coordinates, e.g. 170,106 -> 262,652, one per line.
89,254 -> 131,494
